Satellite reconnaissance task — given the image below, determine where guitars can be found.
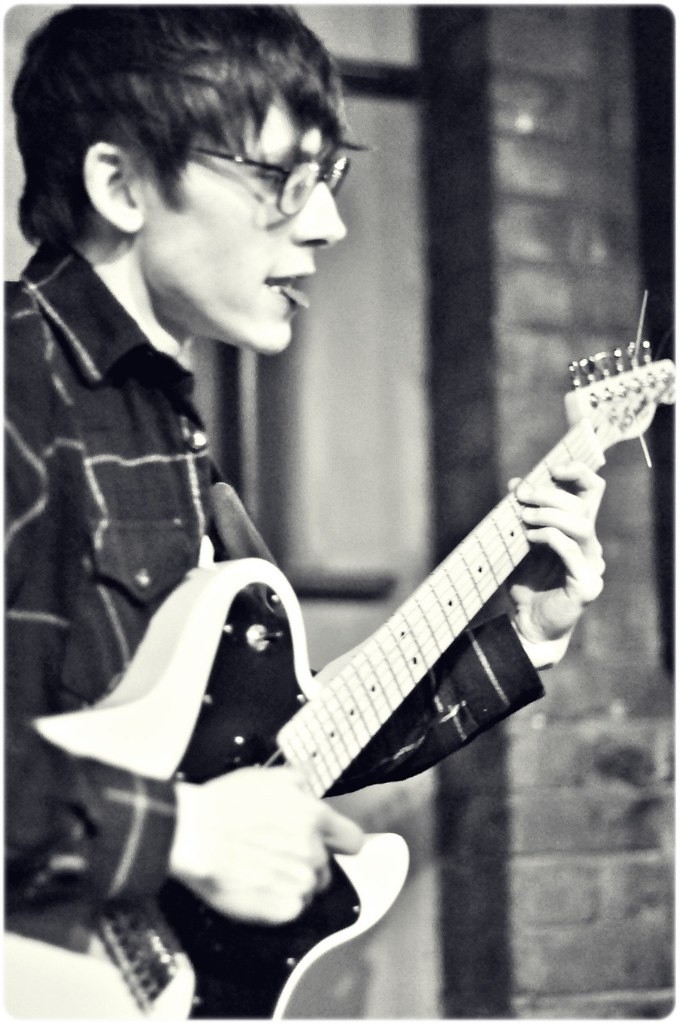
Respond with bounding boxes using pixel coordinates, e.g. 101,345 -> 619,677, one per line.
5,338 -> 676,1021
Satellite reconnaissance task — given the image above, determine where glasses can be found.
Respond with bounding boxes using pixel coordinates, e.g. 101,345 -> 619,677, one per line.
181,126 -> 353,216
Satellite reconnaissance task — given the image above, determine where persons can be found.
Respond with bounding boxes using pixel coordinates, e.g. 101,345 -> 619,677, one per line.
3,5 -> 607,1019
